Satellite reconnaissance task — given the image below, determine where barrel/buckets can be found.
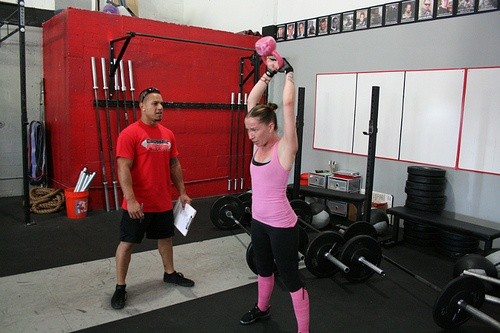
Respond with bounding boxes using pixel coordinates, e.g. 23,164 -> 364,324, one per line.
64,187 -> 90,219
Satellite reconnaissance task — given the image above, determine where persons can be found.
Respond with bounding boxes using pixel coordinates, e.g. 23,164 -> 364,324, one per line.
239,57 -> 309,333
110,88 -> 195,310
276,0 -> 500,41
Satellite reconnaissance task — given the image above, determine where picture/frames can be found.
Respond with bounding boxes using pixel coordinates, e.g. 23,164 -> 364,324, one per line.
274,0 -> 500,43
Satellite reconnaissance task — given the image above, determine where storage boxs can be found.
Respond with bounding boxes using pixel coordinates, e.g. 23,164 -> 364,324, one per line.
308,173 -> 360,193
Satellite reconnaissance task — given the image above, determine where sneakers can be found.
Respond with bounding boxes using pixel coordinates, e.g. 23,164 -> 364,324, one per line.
163,271 -> 195,287
240,303 -> 272,325
111,283 -> 127,310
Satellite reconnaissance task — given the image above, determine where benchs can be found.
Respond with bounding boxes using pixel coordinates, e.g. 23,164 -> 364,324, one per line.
379,207 -> 500,257
284,183 -> 365,223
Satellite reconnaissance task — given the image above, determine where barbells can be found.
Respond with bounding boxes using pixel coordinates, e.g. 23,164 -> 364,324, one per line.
281,183 -> 386,284
236,193 -> 310,263
342,221 -> 500,333
209,194 -> 278,279
